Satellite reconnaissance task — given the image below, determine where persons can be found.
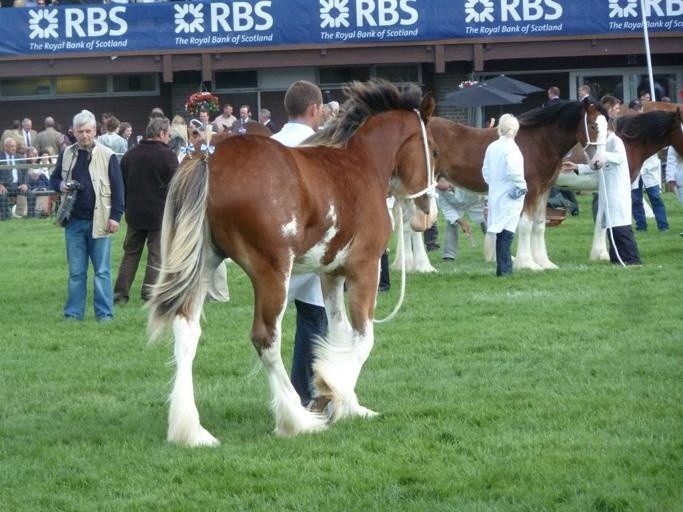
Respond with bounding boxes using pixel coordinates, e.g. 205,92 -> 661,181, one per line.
322,105 -> 334,126
261,78 -> 342,419
436,175 -> 487,260
481,114 -> 529,280
47,108 -> 122,326
548,85 -> 683,231
427,200 -> 440,251
111,114 -> 180,310
1,105 -> 276,221
432,172 -> 488,261
372,247 -> 392,293
586,128 -> 646,270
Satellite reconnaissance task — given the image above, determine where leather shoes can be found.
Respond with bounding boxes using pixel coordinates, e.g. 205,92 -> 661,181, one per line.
571,207 -> 579,216
377,285 -> 389,291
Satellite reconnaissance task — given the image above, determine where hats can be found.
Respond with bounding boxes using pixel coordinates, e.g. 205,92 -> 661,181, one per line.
629,100 -> 642,111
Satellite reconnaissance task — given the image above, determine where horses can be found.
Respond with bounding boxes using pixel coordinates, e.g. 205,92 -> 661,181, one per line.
386,96 -> 610,274
136,75 -> 440,450
481,105 -> 683,264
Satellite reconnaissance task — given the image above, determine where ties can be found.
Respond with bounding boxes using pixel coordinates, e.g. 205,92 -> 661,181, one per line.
243,119 -> 245,124
9,154 -> 14,183
26,131 -> 30,148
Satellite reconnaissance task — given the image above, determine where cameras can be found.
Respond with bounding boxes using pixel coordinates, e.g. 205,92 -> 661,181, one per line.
66,179 -> 86,193
